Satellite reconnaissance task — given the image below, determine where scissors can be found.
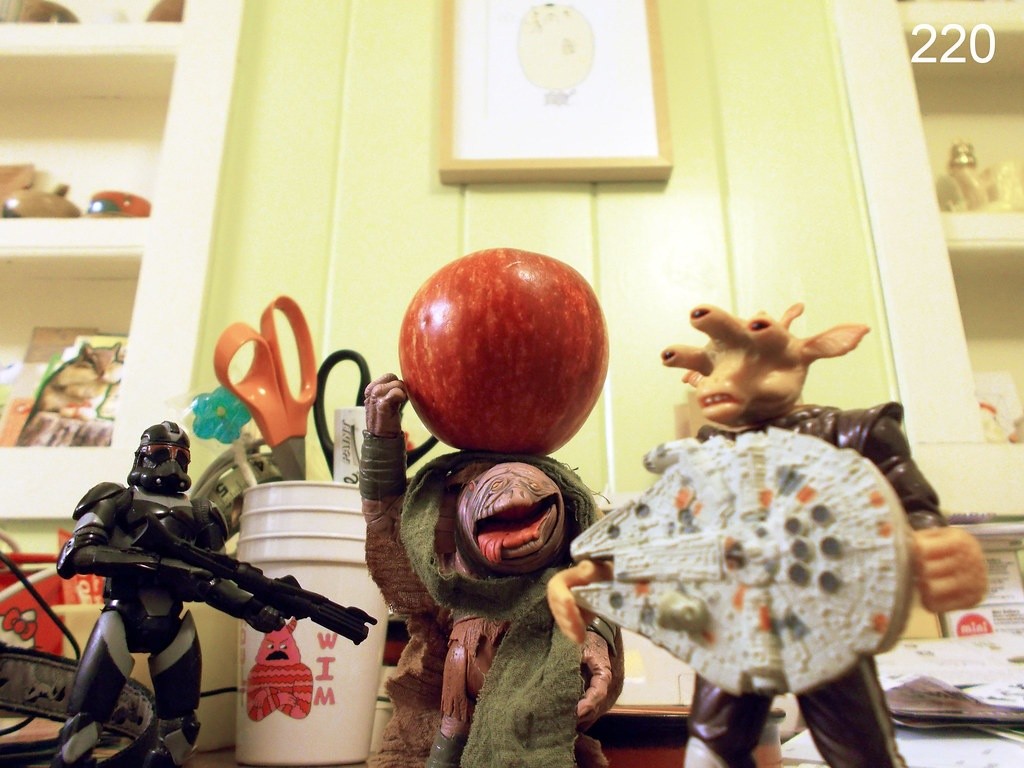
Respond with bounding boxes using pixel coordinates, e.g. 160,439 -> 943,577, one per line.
214,296 -> 318,480
312,349 -> 440,481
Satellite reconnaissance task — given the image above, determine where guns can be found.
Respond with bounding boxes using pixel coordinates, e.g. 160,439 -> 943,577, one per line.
129,511 -> 378,645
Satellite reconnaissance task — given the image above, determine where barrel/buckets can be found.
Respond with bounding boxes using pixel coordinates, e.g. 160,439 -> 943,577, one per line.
2,536 -> 62,660
51,600 -> 238,752
236,479 -> 388,765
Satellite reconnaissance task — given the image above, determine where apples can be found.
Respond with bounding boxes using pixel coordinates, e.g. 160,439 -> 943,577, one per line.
399,248 -> 609,459
